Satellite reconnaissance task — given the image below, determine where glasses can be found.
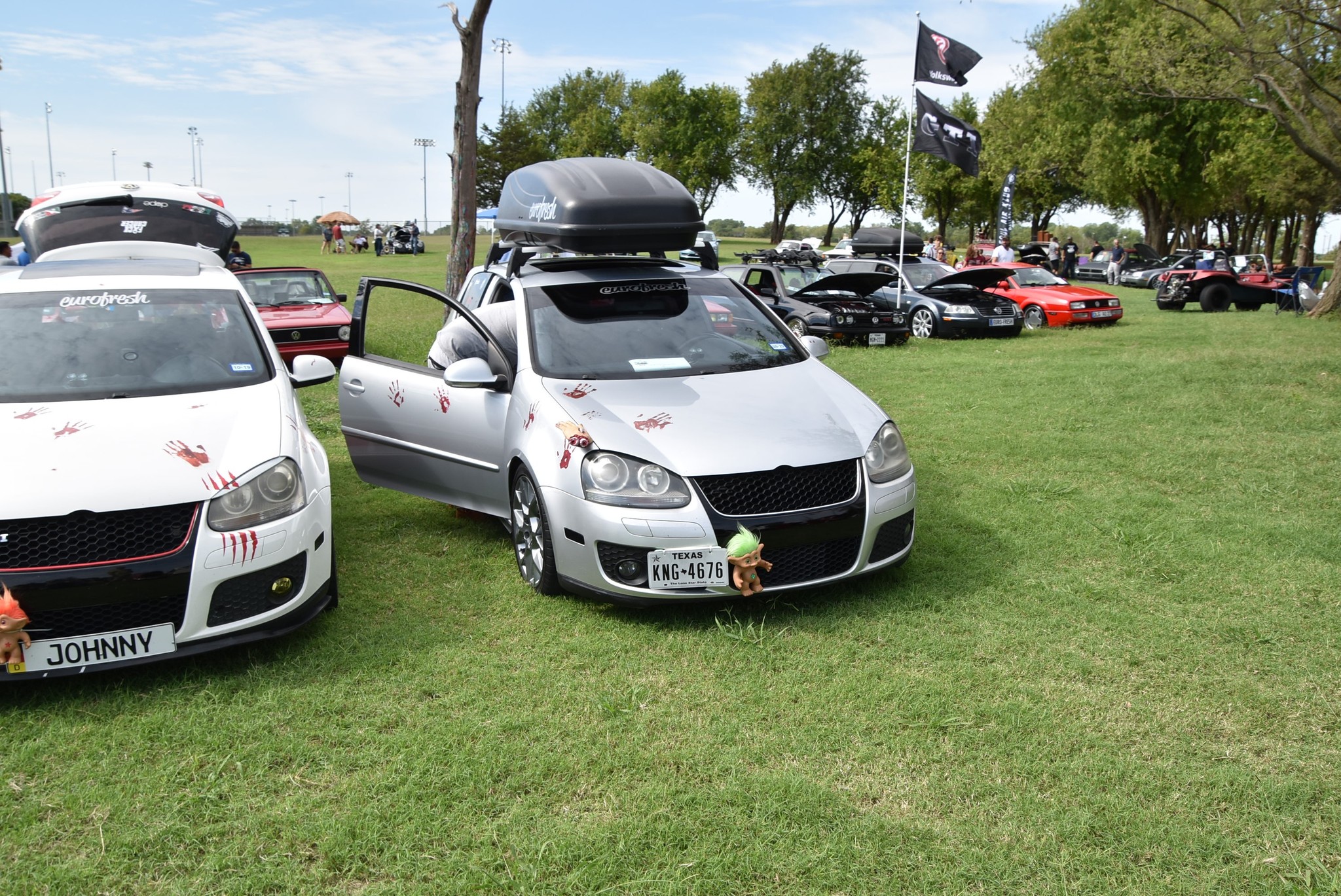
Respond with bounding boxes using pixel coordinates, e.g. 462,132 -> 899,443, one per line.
938,252 -> 944,255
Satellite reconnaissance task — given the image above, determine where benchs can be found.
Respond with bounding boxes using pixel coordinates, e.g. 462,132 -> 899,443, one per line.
245,282 -> 312,298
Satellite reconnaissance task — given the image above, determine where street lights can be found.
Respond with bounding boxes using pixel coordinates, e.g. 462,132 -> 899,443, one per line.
413,139 -> 435,235
112,149 -> 116,182
491,38 -> 511,130
45,102 -> 54,188
187,127 -> 203,188
317,196 -> 324,216
288,200 -> 296,236
144,161 -> 152,182
56,172 -> 66,186
345,172 -> 354,235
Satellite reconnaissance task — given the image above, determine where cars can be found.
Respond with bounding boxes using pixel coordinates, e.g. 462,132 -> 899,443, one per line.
1077,244 -> 1311,313
679,231 -> 721,261
385,227 -> 424,255
1,182 -> 354,683
703,237 -> 1126,346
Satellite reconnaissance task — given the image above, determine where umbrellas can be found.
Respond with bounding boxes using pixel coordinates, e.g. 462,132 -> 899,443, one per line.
476,208 -> 499,243
316,211 -> 361,226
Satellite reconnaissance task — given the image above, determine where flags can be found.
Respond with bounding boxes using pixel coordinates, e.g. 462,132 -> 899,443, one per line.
911,89 -> 982,180
994,164 -> 1019,247
914,19 -> 983,86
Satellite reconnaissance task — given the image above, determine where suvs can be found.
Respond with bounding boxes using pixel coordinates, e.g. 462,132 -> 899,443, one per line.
341,157 -> 918,605
814,226 -> 1025,340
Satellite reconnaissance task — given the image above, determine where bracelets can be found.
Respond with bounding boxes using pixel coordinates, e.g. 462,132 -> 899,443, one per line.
238,265 -> 241,269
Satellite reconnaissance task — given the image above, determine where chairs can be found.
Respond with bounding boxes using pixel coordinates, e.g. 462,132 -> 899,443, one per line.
1271,265 -> 1326,318
285,279 -> 311,298
1248,263 -> 1256,273
788,277 -> 801,289
239,280 -> 263,304
1274,264 -> 1286,273
1258,263 -> 1270,274
1271,263 -> 1277,269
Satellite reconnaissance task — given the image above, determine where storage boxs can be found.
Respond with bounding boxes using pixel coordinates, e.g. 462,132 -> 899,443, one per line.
488,152 -> 707,261
848,226 -> 926,257
1037,230 -> 1053,244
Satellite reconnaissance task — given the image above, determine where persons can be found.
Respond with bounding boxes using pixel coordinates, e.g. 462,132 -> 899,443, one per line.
409,221 -> 419,256
1217,242 -> 1238,256
332,222 -> 349,254
991,235 -> 1015,263
1089,239 -> 1105,262
374,224 -> 384,256
320,223 -> 333,255
1047,237 -> 1061,275
1107,239 -> 1125,285
349,233 -> 367,254
0,241 -> 19,266
1062,236 -> 1080,281
17,247 -> 32,266
225,240 -> 252,272
426,298 -> 553,374
922,235 -> 986,268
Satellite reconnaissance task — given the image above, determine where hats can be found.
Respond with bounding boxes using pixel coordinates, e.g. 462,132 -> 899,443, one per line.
1002,235 -> 1010,240
1067,236 -> 1072,240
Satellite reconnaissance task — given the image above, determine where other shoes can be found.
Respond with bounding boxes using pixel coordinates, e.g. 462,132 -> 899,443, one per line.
456,507 -> 472,516
